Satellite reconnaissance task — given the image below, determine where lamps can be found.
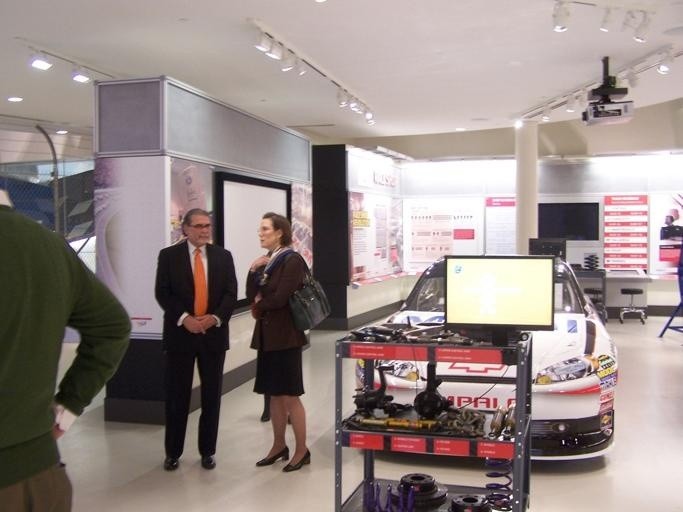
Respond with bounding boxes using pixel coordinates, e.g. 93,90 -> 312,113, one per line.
503,88 -> 583,135
574,46 -> 678,99
552,1 -> 659,45
247,16 -> 328,85
331,75 -> 380,126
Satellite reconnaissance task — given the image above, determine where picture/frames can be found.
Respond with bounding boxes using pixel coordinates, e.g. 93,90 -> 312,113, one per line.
209,169 -> 294,316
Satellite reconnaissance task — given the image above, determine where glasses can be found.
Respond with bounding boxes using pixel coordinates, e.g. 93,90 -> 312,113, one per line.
188,224 -> 211,229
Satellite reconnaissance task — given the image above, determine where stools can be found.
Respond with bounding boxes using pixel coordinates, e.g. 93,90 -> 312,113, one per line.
583,286 -> 608,323
618,287 -> 648,325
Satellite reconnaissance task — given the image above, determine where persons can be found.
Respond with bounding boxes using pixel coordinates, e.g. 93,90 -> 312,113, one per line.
664,209 -> 680,227
243,212 -> 317,474
0,203 -> 134,511
151,208 -> 237,471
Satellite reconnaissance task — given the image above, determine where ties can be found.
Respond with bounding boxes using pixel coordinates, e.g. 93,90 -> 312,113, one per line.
193,247 -> 209,317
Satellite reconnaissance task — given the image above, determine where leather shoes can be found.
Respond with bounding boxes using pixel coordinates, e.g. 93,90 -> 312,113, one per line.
164,457 -> 179,471
201,456 -> 216,469
261,411 -> 270,422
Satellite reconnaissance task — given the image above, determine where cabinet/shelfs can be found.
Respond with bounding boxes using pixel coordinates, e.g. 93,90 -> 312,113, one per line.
331,330 -> 534,512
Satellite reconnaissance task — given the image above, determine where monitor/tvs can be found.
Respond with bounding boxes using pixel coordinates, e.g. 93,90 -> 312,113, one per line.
528,237 -> 566,261
444,254 -> 556,344
538,203 -> 600,243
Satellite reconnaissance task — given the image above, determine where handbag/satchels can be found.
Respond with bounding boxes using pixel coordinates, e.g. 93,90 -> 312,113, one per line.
288,252 -> 332,332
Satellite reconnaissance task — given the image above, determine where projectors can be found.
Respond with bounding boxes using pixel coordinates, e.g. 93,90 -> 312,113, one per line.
582,101 -> 636,126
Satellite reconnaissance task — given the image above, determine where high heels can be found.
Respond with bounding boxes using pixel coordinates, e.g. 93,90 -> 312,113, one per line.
256,445 -> 290,466
283,448 -> 311,472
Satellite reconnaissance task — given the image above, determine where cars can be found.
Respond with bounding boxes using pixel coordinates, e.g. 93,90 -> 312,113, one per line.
355,253 -> 619,464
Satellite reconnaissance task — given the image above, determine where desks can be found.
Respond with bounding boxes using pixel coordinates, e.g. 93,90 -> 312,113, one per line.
574,268 -> 606,321
